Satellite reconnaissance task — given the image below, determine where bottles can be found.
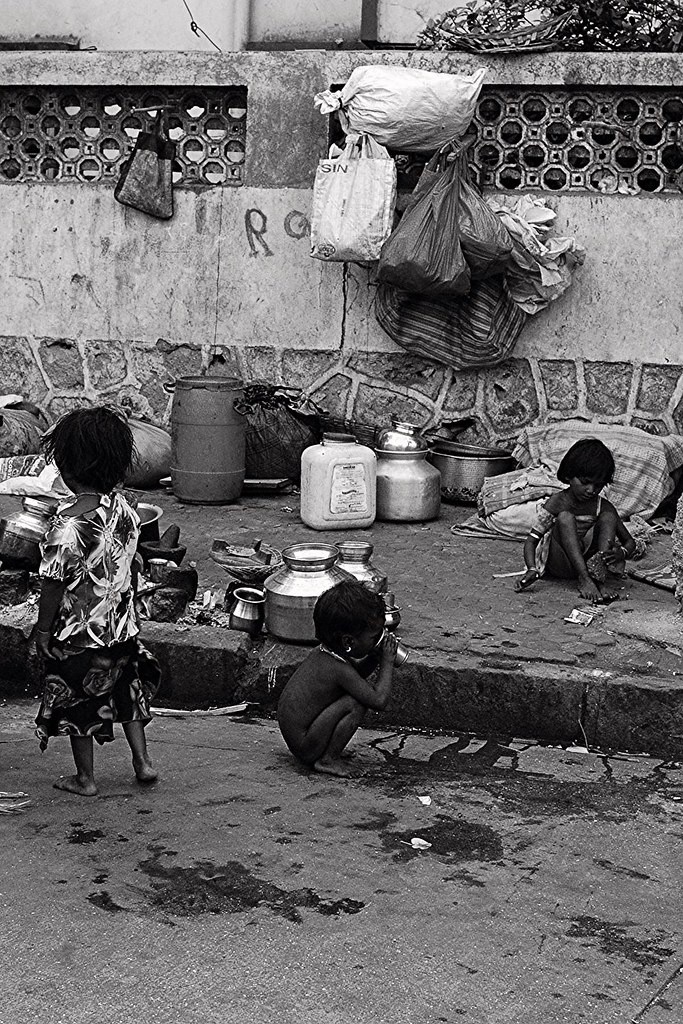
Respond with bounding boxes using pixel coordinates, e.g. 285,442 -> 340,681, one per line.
228,540 -> 401,646
375,420 -> 443,523
0,496 -> 58,570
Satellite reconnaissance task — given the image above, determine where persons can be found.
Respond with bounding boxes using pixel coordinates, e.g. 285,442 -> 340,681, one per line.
514,436 -> 636,603
36,405 -> 160,795
277,578 -> 399,779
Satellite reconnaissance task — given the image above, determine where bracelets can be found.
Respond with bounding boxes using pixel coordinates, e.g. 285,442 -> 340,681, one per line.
37,629 -> 48,635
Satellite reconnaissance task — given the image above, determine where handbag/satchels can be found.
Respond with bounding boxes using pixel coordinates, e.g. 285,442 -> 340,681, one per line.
376,140 -> 529,369
311,131 -> 396,262
115,109 -> 177,221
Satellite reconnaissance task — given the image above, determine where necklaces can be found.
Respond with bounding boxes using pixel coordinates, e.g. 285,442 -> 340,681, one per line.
319,644 -> 347,663
75,493 -> 100,498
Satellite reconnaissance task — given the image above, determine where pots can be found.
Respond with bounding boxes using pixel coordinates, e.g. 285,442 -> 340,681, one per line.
428,444 -> 517,505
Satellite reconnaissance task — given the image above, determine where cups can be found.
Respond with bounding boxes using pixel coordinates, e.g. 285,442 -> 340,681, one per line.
371,628 -> 410,668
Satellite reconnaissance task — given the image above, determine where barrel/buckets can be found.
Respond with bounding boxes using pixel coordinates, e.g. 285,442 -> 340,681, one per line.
161,372 -> 255,506
297,431 -> 377,531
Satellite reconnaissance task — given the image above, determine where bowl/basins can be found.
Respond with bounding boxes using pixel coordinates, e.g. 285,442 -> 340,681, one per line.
135,502 -> 164,552
